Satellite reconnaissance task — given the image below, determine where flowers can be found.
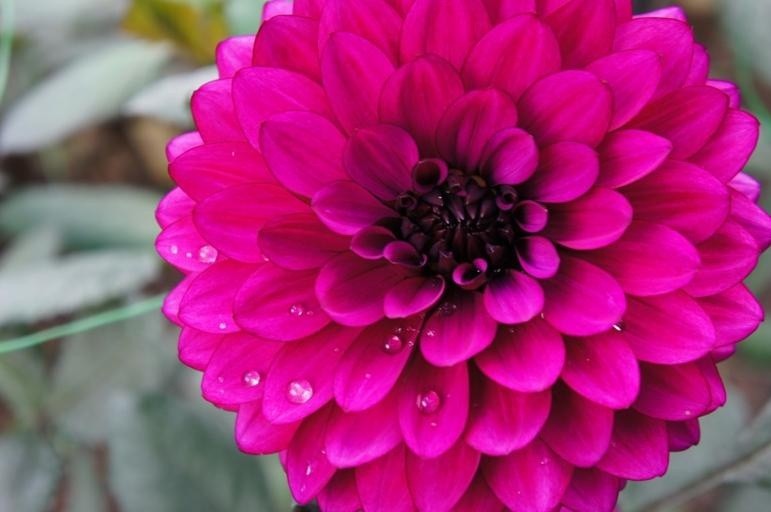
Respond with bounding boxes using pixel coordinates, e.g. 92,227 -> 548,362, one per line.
154,2 -> 771,511
